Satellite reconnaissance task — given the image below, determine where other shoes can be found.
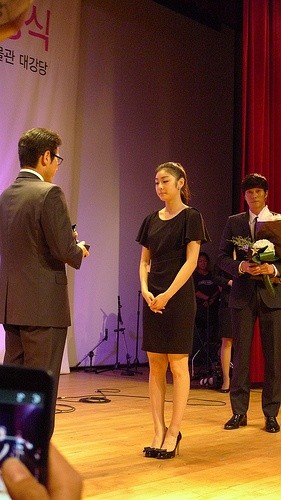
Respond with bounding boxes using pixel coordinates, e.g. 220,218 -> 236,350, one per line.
220,386 -> 230,393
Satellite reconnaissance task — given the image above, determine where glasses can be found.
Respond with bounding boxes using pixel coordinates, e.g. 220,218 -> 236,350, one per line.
42,150 -> 63,165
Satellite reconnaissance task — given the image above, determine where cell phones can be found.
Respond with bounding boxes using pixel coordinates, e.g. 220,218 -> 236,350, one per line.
0,364 -> 57,500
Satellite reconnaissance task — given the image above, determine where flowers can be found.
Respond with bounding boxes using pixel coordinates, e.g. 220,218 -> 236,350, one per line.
226,236 -> 276,299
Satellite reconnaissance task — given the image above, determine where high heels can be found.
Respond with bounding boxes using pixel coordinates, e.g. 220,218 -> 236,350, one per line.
156,430 -> 182,459
142,427 -> 168,458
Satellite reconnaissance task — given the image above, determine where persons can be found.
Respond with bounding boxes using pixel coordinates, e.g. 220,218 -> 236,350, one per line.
136,161 -> 211,460
193,252 -> 237,393
1,126 -> 91,441
216,173 -> 281,433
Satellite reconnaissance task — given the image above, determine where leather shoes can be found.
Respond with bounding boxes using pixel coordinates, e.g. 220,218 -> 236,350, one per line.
224,414 -> 247,429
265,416 -> 280,433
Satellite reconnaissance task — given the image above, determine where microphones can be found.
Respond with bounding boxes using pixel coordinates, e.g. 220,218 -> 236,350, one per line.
105,329 -> 109,341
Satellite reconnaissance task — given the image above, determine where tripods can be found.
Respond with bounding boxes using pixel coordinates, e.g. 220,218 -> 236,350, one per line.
95,296 -> 143,376
192,300 -> 224,379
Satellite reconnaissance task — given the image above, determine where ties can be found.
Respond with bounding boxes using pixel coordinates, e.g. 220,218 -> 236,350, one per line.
254,217 -> 258,242
